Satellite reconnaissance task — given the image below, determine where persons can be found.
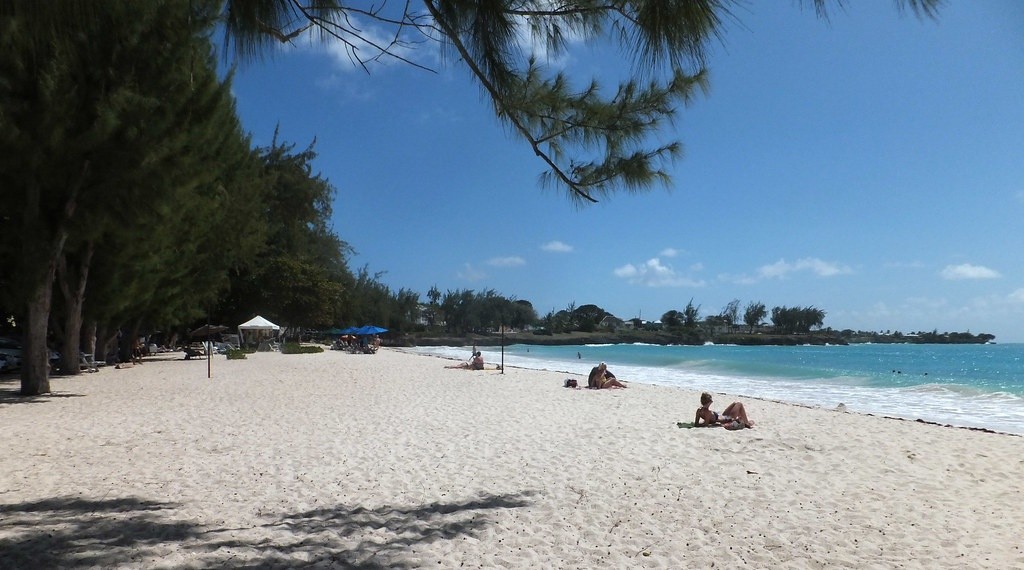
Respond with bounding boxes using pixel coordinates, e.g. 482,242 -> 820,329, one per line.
694,393 -> 755,426
373,335 -> 380,351
444,343 -> 484,370
340,335 -> 363,350
132,335 -> 144,365
363,335 -> 368,351
263,333 -> 270,340
187,345 -> 202,356
259,333 -> 264,342
248,332 -> 253,345
589,363 -> 626,389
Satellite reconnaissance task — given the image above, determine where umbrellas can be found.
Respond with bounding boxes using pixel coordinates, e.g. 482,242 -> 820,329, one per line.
357,325 -> 389,337
339,328 -> 359,334
191,324 -> 228,335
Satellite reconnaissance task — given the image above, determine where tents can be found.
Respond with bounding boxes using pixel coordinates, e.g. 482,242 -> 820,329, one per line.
237,315 -> 279,344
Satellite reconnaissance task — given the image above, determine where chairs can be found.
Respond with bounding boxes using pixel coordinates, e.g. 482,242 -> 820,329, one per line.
184,348 -> 213,359
47,351 -> 106,372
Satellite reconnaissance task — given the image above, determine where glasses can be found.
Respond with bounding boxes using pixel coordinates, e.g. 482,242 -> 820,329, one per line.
710,400 -> 713,403
